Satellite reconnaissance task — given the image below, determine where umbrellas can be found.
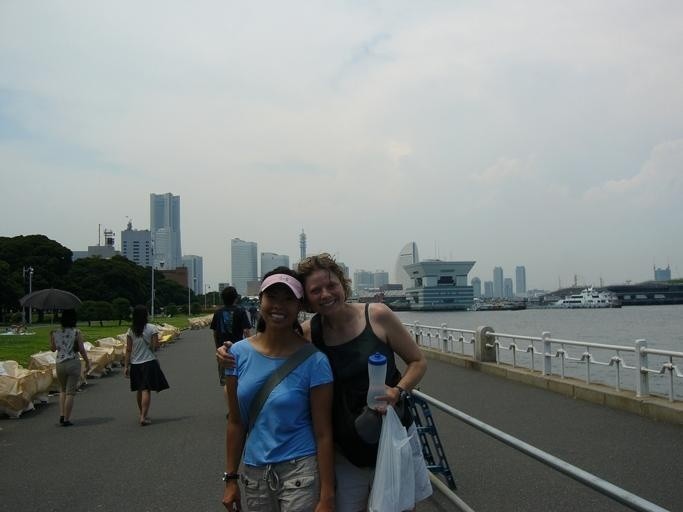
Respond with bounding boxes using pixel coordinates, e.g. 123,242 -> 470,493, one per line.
20,287 -> 83,332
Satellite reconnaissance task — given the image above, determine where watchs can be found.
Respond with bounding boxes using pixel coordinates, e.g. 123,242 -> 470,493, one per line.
222,472 -> 241,481
395,385 -> 410,406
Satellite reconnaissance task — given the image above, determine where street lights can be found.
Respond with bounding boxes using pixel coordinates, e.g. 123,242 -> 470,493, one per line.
186,272 -> 196,318
149,240 -> 155,321
23,266 -> 34,323
205,283 -> 211,309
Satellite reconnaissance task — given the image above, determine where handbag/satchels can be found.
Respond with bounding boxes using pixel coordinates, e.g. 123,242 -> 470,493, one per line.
73,340 -> 79,353
355,397 -> 412,444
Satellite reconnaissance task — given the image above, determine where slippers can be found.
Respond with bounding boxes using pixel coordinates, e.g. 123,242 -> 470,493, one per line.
139,417 -> 152,426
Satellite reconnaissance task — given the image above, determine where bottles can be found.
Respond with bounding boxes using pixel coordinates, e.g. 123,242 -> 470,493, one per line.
366,352 -> 387,410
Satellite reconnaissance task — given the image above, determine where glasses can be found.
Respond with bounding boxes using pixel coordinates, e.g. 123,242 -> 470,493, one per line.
300,253 -> 330,265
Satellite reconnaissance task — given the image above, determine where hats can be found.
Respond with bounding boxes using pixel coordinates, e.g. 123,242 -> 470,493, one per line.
259,273 -> 303,301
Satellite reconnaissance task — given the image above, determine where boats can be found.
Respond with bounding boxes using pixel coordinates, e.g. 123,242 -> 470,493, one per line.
472,300 -> 526,311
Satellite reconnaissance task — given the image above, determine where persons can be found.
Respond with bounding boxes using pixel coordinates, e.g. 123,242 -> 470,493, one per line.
209,286 -> 251,424
49,309 -> 91,426
215,252 -> 428,512
123,304 -> 170,425
221,265 -> 336,512
248,302 -> 257,328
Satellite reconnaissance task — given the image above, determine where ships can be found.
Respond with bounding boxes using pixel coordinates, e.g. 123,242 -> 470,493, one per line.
551,286 -> 621,309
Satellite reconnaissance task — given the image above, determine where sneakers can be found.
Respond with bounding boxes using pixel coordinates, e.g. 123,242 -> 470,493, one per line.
252,325 -> 255,328
62,420 -> 72,426
59,415 -> 63,423
